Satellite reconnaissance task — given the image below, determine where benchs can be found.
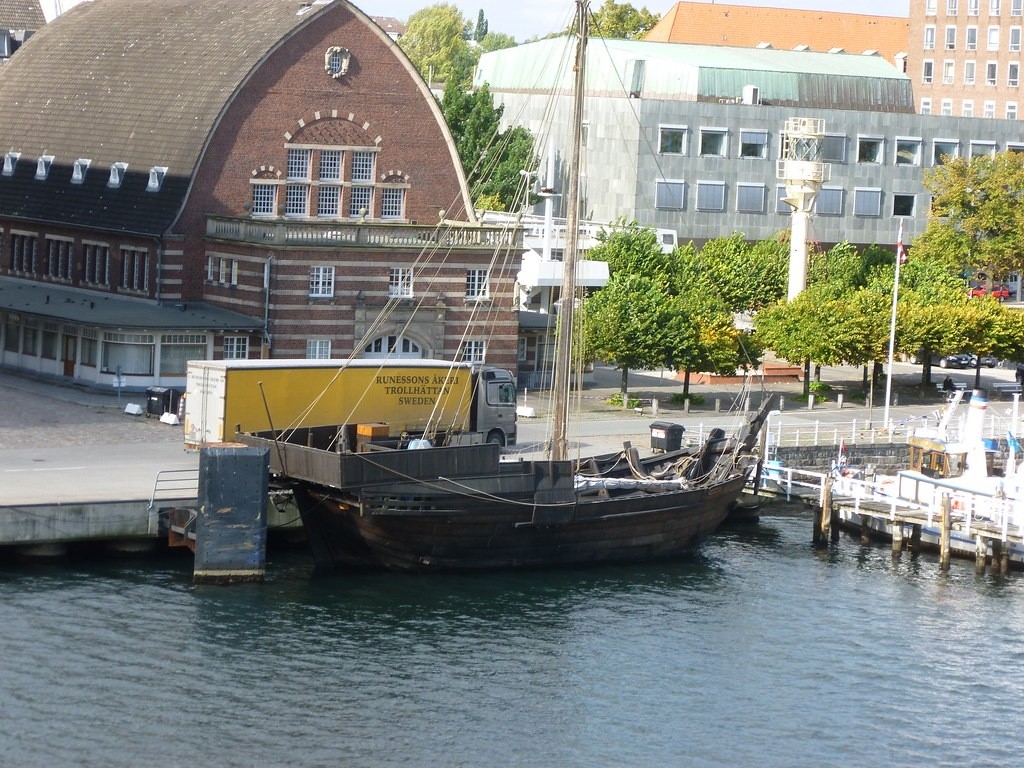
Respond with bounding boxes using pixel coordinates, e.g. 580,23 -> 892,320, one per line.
993,383 -> 1023,399
936,383 -> 973,398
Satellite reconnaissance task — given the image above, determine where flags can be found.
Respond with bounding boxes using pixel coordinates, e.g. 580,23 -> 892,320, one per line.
841,440 -> 846,452
897,224 -> 906,264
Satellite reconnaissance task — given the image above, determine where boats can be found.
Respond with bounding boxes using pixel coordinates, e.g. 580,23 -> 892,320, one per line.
831,386 -> 1024,527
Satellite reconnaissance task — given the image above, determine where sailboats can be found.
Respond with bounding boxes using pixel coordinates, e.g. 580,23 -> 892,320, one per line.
237,0 -> 776,573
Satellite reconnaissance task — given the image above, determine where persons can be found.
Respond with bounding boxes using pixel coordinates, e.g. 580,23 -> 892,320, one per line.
944,375 -> 956,397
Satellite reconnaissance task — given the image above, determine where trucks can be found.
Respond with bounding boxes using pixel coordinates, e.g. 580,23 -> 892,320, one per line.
184,358 -> 518,451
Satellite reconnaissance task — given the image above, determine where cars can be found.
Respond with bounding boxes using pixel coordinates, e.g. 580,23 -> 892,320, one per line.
1015,361 -> 1024,384
967,281 -> 1009,302
910,346 -> 998,369
